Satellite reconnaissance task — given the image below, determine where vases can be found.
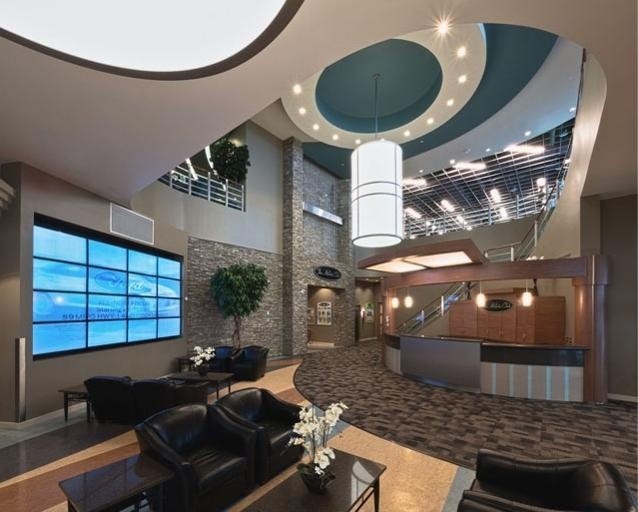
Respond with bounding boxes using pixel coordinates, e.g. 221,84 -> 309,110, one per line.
299,459 -> 336,491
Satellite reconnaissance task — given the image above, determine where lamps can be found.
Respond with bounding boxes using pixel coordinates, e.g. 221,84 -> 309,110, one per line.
349,74 -> 404,248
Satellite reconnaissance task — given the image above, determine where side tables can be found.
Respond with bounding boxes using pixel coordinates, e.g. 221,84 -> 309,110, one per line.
59,453 -> 173,512
177,353 -> 198,372
58,383 -> 91,424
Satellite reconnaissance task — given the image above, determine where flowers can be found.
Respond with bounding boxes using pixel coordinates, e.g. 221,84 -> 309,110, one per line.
287,403 -> 347,471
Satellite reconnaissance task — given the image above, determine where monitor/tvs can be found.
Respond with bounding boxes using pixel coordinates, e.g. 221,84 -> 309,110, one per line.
32,212 -> 184,361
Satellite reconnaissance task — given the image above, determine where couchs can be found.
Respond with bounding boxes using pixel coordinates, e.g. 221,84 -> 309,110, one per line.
135,404 -> 258,512
214,386 -> 307,483
228,346 -> 269,381
200,346 -> 235,375
85,373 -> 208,425
459,447 -> 636,511
